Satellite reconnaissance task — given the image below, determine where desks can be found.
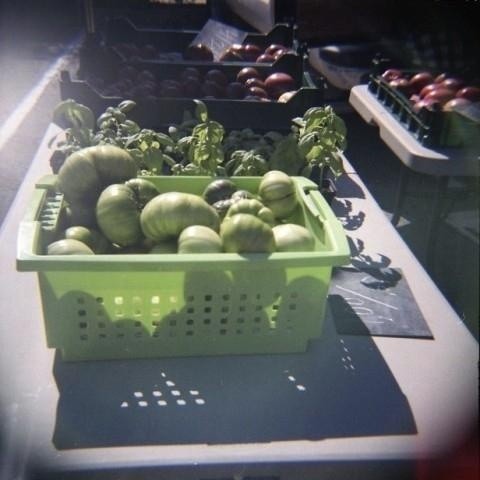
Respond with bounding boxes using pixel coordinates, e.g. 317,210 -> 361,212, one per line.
0,39 -> 479,480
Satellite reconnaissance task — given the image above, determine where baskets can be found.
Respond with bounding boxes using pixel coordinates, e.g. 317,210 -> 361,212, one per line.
16,174 -> 352,362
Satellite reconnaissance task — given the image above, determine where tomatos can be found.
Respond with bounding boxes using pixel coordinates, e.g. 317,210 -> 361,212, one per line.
43,143 -> 316,256
382,68 -> 480,113
80,40 -> 299,104
